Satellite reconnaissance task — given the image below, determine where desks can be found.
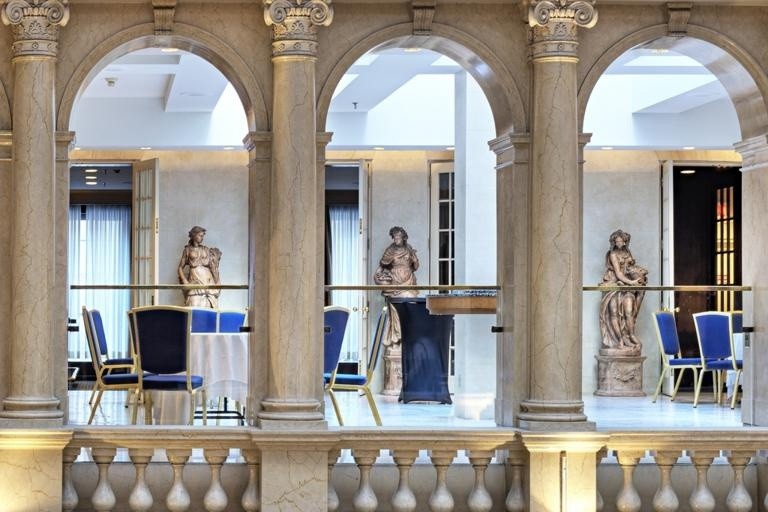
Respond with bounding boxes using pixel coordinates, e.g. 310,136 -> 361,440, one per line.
389,297 -> 454,404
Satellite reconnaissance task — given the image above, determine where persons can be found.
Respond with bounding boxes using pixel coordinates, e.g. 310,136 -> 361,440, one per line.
178,223 -> 221,309
597,228 -> 648,350
373,227 -> 419,354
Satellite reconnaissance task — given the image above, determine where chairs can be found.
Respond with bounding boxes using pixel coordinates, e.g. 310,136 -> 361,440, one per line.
651,310 -> 744,410
